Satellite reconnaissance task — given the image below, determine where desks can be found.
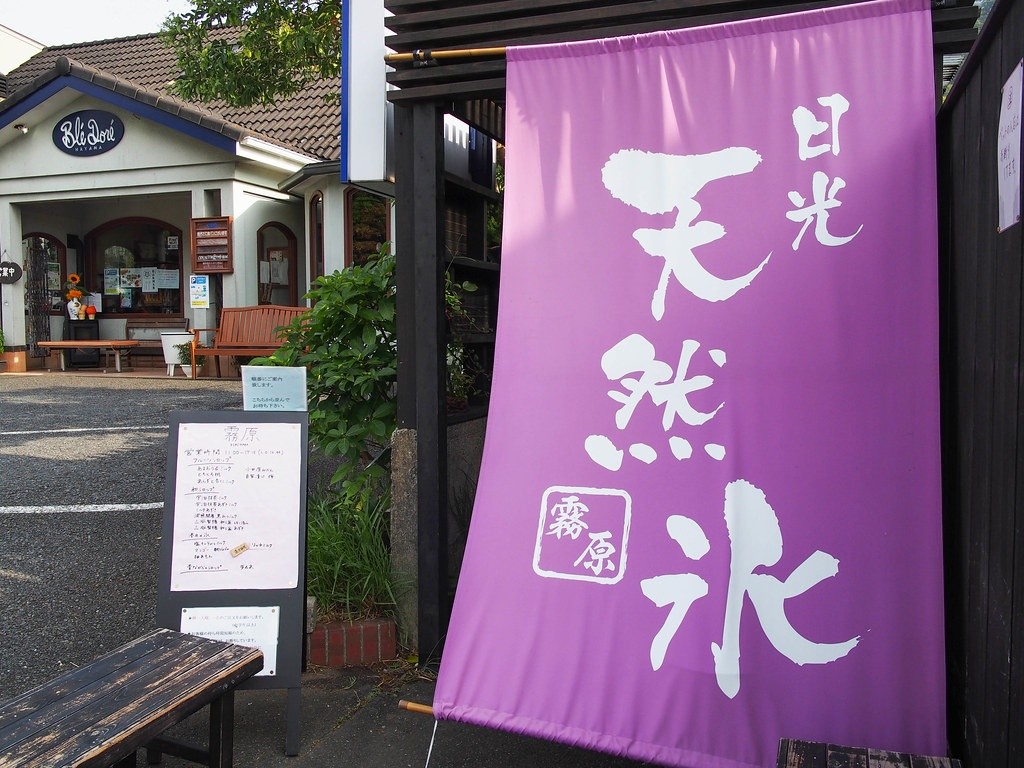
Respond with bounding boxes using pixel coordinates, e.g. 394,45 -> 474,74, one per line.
161,331 -> 195,376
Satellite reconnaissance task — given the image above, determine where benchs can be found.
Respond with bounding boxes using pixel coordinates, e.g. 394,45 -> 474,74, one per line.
188,304 -> 313,380
0,628 -> 263,768
105,318 -> 190,368
38,340 -> 139,374
775,738 -> 960,768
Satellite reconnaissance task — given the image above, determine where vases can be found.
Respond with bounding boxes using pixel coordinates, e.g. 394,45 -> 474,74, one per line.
67,297 -> 81,320
180,364 -> 204,377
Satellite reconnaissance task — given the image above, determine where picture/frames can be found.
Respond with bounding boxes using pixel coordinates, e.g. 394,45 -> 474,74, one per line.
267,247 -> 289,288
51,296 -> 61,311
44,262 -> 61,290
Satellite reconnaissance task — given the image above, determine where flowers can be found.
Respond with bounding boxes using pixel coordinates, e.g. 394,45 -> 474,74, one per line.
64,273 -> 82,301
173,341 -> 211,367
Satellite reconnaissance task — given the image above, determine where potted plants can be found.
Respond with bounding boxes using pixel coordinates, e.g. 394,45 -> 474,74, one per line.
0,325 -> 7,372
232,355 -> 264,374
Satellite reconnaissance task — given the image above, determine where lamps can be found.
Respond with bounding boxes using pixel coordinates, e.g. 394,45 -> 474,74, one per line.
18,126 -> 28,134
